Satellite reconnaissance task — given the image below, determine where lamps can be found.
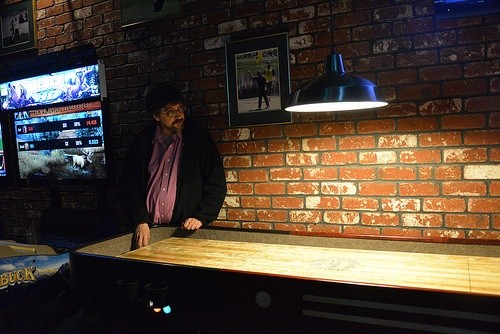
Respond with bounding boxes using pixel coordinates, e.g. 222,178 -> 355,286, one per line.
284,0 -> 389,112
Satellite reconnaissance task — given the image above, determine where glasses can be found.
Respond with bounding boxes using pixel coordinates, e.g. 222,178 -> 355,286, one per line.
156,104 -> 187,117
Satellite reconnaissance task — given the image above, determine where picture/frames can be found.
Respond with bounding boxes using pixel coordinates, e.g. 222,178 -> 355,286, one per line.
223,32 -> 294,127
119,0 -> 182,29
0,1 -> 38,57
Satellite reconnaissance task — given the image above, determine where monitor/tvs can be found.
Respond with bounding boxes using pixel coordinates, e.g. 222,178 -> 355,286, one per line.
0,59 -> 109,184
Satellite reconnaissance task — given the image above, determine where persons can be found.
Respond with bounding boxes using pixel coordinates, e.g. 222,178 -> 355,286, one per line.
125,86 -> 226,249
248,71 -> 270,110
262,60 -> 276,102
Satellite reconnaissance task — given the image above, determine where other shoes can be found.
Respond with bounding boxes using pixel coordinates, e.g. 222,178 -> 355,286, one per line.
256,106 -> 271,110
268,97 -> 271,102
